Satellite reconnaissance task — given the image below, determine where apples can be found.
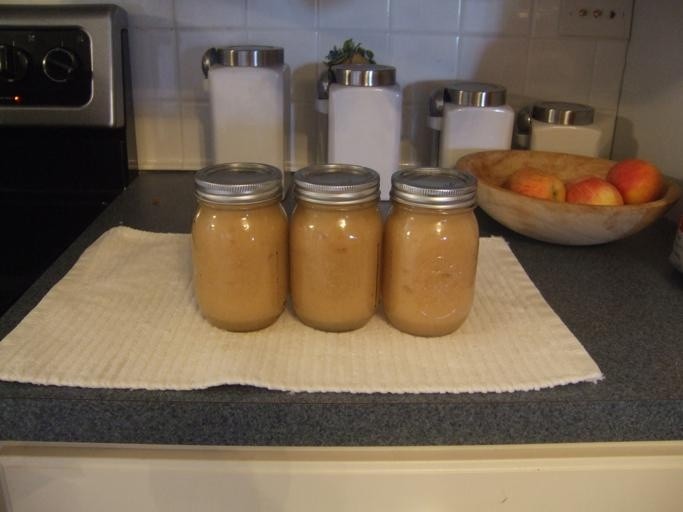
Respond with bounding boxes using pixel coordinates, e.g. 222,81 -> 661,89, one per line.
510,166 -> 566,203
607,159 -> 664,205
566,177 -> 624,205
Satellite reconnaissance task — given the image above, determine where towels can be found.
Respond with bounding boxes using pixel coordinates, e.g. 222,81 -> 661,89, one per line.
1,225 -> 606,394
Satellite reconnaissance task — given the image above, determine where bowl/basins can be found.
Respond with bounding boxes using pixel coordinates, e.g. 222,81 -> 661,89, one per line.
457,150 -> 681,249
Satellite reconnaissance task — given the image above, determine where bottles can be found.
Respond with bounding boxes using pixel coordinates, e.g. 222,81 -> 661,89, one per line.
313,46 -> 404,202
203,44 -> 288,202
426,79 -> 600,167
